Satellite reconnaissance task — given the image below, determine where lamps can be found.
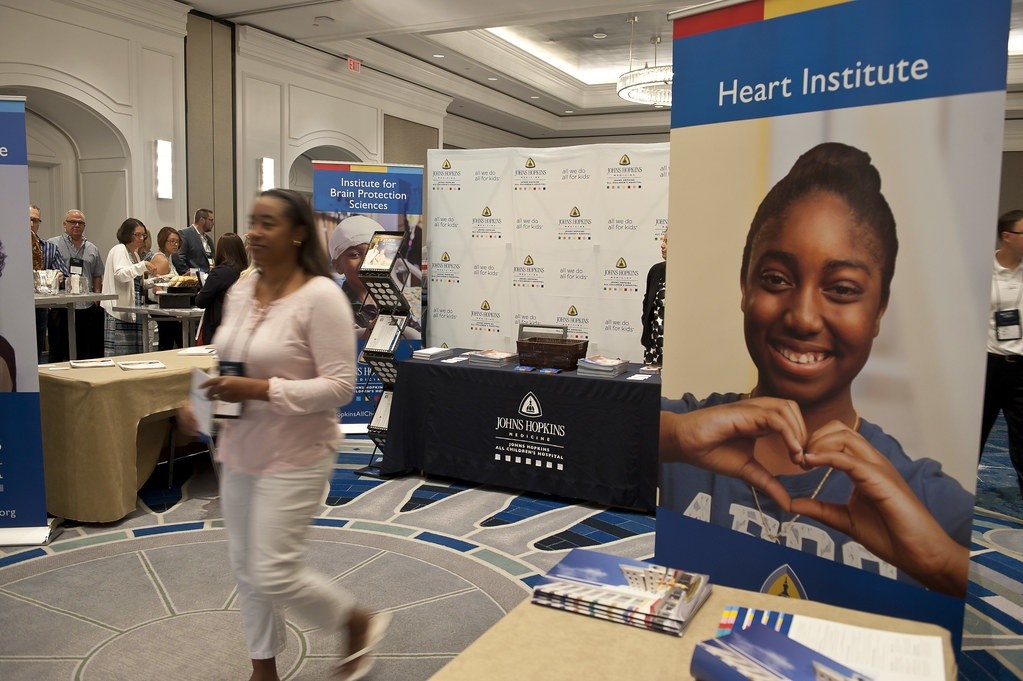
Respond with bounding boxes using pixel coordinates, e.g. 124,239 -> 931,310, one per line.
256,156 -> 274,192
617,10 -> 674,106
151,139 -> 173,199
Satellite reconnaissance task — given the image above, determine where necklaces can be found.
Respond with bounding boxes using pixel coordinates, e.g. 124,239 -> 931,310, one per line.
750,393 -> 859,540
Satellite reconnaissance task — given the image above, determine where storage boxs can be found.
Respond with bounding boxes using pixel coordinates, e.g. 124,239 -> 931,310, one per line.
516,337 -> 589,371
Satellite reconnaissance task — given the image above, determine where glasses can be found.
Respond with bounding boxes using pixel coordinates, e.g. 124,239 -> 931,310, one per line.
167,239 -> 180,244
30,217 -> 41,223
209,218 -> 214,221
130,233 -> 147,238
66,220 -> 85,225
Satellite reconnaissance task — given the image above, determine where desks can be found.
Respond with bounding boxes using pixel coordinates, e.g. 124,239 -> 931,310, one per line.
111,305 -> 203,354
379,348 -> 661,514
425,585 -> 963,681
34,292 -> 119,362
35,342 -> 216,523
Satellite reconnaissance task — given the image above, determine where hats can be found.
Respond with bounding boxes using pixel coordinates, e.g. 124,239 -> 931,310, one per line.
329,215 -> 386,260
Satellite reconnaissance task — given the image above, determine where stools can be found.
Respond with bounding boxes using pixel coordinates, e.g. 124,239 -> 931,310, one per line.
168,415 -> 218,494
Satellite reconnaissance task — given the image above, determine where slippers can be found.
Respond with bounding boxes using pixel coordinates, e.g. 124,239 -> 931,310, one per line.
335,607 -> 394,681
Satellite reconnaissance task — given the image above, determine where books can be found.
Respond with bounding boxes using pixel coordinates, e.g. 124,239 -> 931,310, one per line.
413,347 -> 629,378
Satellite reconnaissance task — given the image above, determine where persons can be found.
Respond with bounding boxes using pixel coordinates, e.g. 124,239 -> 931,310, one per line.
328,217 -> 385,338
640,228 -> 668,367
0,244 -> 17,395
30,204 -> 346,359
178,188 -> 389,681
657,142 -> 973,597
974,210 -> 1023,493
